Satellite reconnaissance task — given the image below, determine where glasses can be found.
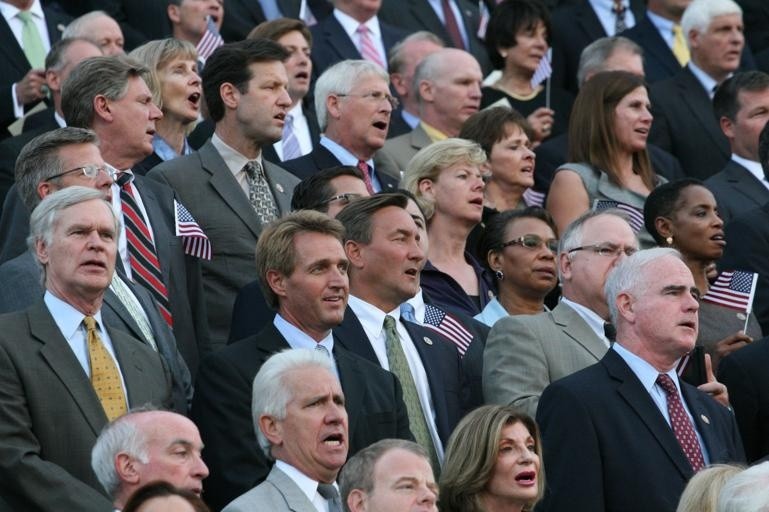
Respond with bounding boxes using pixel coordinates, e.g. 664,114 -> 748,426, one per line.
45,164 -> 113,182
500,235 -> 558,254
337,90 -> 400,111
320,194 -> 361,204
568,245 -> 639,257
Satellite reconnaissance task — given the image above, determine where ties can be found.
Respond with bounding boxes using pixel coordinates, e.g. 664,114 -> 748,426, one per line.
15,11 -> 51,101
605,324 -> 615,347
383,303 -> 440,484
84,317 -> 128,422
357,23 -> 384,68
357,161 -> 374,196
318,483 -> 344,512
671,26 -> 692,68
612,1 -> 627,34
243,160 -> 280,225
111,172 -> 173,352
441,0 -> 465,50
656,374 -> 705,473
282,115 -> 303,162
